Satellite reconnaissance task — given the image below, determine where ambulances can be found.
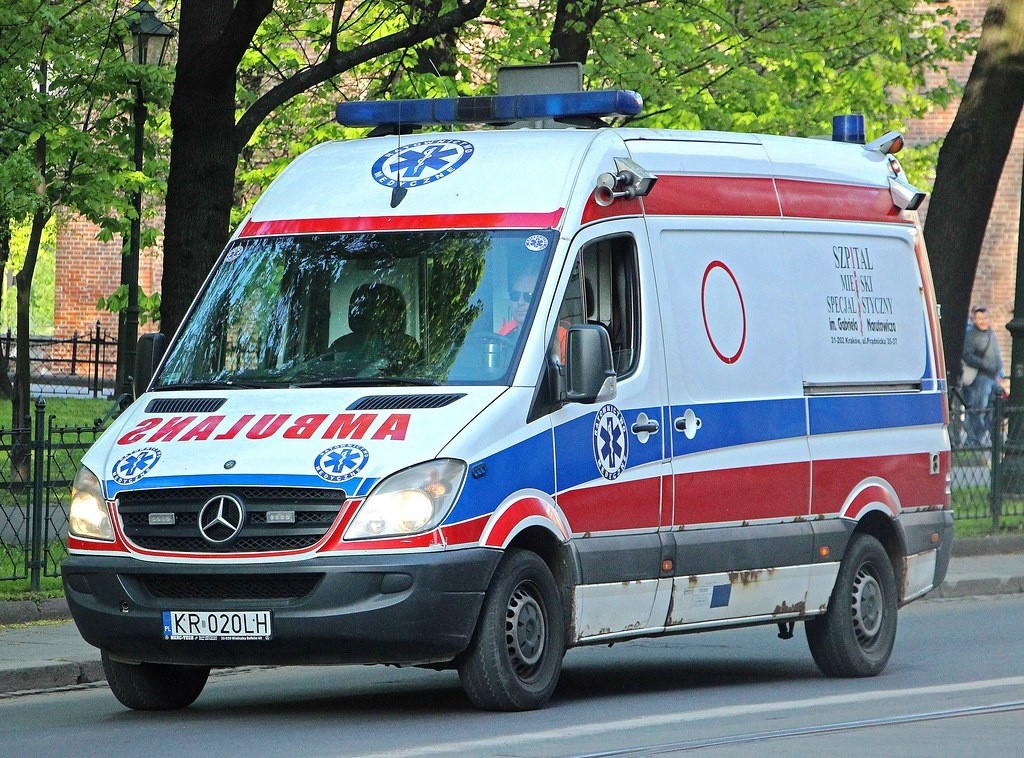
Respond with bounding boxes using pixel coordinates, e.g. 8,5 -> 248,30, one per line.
62,61 -> 955,709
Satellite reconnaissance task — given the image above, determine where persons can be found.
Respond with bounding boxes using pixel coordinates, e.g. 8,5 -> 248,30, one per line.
494,263 -> 568,387
961,305 -> 1003,450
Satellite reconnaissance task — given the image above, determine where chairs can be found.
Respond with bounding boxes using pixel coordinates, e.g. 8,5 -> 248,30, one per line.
323,283 -> 422,370
561,277 -> 611,338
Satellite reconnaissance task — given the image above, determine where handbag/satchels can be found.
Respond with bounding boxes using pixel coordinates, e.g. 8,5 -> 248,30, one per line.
961,362 -> 978,385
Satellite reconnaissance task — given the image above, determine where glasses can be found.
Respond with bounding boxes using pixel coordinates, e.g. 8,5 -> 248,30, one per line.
509,290 -> 534,304
